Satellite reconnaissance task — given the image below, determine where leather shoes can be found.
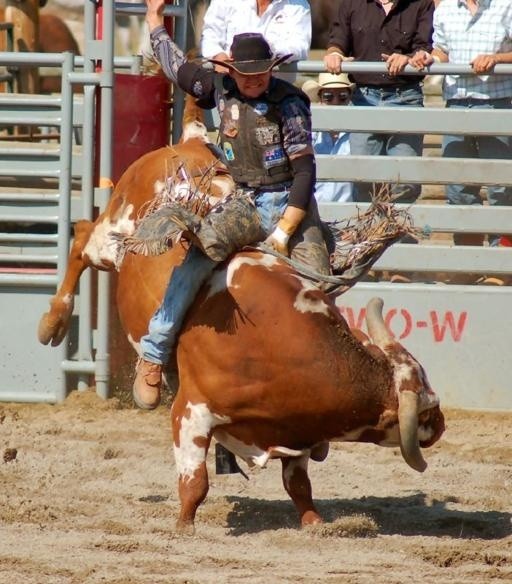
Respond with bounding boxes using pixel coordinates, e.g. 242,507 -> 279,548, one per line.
390,271 -> 411,283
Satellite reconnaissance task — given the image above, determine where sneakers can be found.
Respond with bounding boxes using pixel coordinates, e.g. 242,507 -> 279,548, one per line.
132,357 -> 162,411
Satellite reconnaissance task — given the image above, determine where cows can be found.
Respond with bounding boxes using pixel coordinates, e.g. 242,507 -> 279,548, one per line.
36,47 -> 446,538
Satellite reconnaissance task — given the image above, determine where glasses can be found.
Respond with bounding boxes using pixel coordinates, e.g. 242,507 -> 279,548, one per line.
322,90 -> 349,104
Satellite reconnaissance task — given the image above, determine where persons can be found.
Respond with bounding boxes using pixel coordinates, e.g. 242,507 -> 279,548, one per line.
201,0 -> 313,83
131,0 -> 332,409
302,73 -> 358,274
406,0 -> 511,286
325,0 -> 435,284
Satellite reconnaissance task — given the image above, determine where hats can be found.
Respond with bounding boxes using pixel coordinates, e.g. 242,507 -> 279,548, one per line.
209,31 -> 295,78
301,71 -> 357,103
